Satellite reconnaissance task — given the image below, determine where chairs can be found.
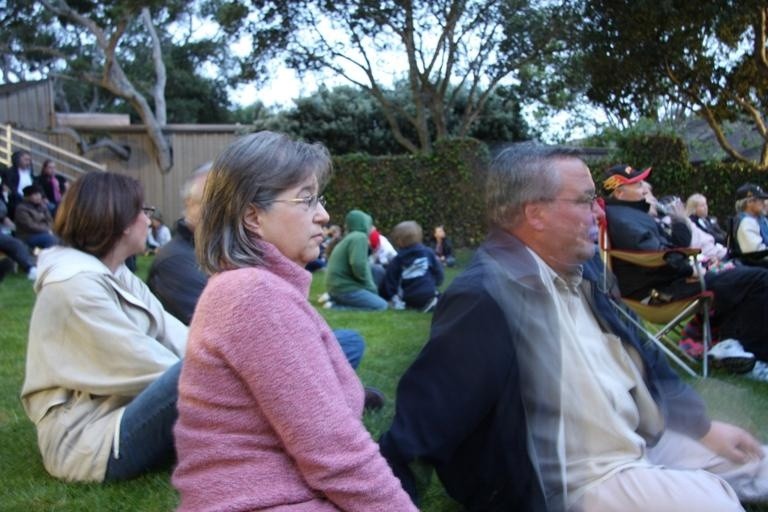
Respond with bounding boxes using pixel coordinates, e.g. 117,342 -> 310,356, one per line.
596,196 -> 713,380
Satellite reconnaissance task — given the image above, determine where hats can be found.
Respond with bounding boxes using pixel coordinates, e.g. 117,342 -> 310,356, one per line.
736,182 -> 768,199
601,163 -> 653,195
23,186 -> 39,195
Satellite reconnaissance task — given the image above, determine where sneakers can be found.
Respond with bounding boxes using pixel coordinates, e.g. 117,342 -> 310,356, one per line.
708,338 -> 756,373
744,361 -> 768,380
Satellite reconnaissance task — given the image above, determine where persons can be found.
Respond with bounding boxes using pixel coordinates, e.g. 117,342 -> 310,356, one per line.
305,210 -> 457,313
148,159 -> 386,408
125,255 -> 137,272
0,151 -> 70,280
19,172 -> 190,483
144,207 -> 172,253
592,161 -> 768,380
370,141 -> 768,511
172,130 -> 417,512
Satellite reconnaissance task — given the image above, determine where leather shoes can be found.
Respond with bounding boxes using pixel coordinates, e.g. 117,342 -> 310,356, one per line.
363,388 -> 384,409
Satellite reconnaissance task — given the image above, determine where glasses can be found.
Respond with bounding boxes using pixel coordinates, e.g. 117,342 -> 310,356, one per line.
271,194 -> 327,207
561,195 -> 598,209
142,206 -> 156,216
